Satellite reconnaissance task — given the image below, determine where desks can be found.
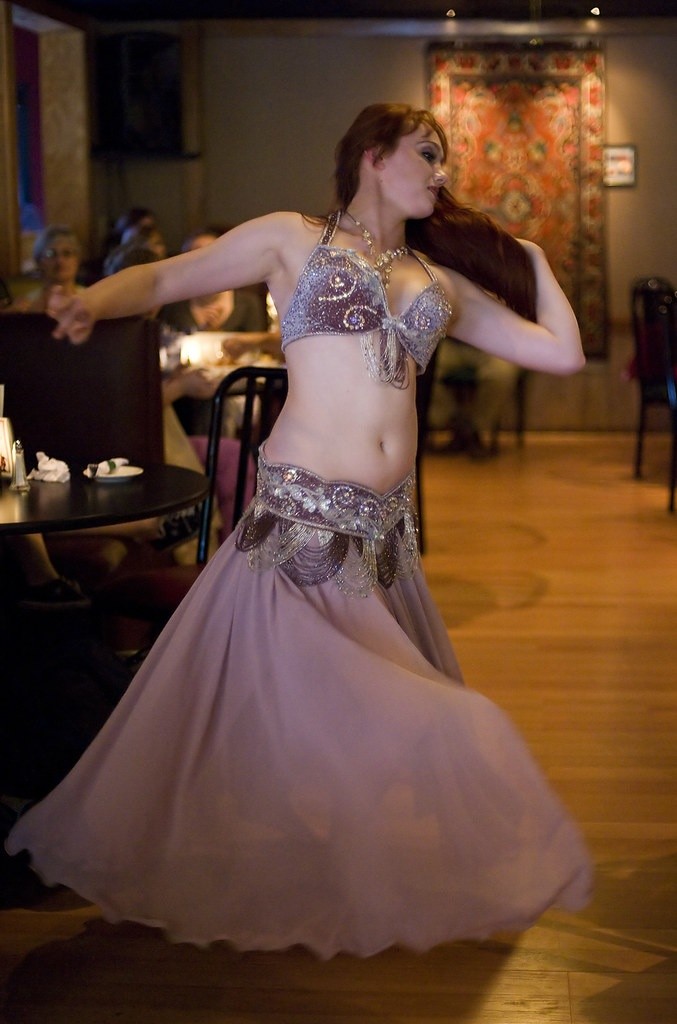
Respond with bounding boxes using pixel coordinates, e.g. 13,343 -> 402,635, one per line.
0,445 -> 212,915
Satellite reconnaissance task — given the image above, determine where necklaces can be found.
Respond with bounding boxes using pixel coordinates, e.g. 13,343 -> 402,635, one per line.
340,209 -> 407,284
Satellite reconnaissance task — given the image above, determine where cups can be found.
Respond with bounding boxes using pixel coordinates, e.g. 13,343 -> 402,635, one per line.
164,332 -> 181,366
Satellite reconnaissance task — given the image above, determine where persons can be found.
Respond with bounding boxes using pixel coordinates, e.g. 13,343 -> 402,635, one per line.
31,222 -> 82,289
5,103 -> 584,962
100,203 -> 271,437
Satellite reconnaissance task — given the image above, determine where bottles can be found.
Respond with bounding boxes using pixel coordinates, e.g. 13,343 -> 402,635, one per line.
9,441 -> 31,490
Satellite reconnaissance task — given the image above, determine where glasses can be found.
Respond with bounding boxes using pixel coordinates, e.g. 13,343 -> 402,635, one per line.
41,248 -> 78,259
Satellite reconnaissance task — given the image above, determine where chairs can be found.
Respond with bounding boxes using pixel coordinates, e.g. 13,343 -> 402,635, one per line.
51,363 -> 289,770
0,310 -> 163,653
630,276 -> 677,513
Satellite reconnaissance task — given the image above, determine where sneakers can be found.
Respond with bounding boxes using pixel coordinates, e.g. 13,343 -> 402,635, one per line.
19,579 -> 91,609
149,512 -> 213,552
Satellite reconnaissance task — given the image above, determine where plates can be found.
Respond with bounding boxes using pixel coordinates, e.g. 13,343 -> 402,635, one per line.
84,466 -> 144,483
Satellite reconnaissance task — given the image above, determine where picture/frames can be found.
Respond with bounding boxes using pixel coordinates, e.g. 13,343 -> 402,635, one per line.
599,143 -> 638,190
